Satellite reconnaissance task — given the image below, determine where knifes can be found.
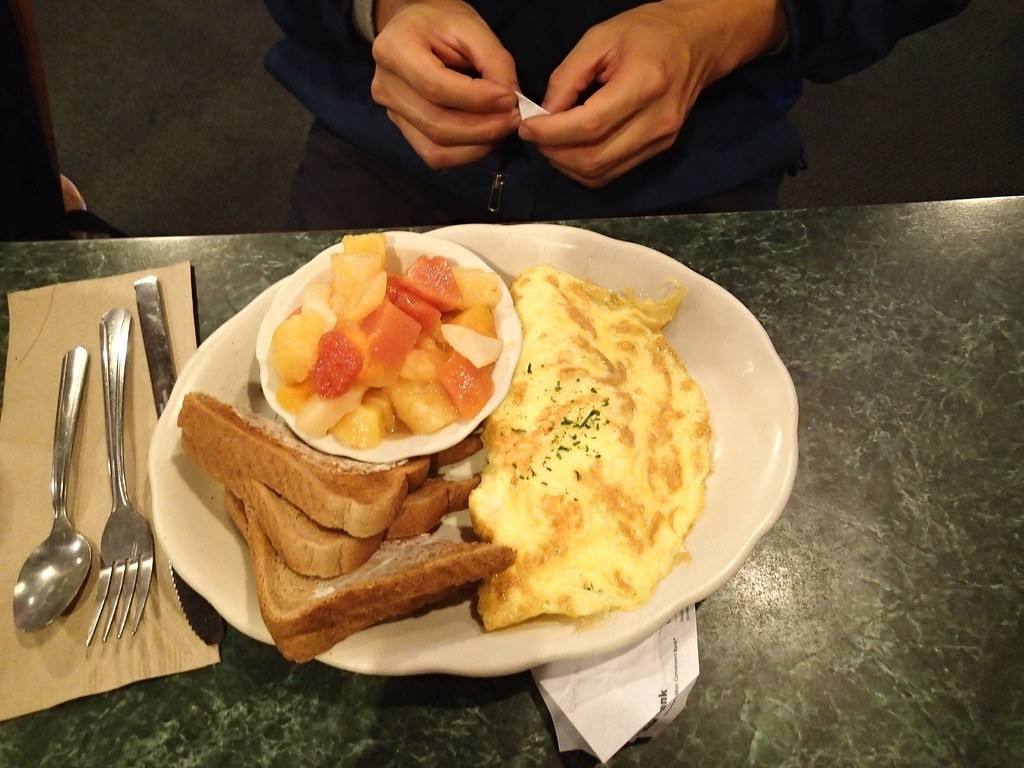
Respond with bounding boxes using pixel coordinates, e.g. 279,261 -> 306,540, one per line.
134,276 -> 225,647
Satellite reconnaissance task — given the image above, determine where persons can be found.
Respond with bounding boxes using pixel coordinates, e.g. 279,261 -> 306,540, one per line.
250,0 -> 970,231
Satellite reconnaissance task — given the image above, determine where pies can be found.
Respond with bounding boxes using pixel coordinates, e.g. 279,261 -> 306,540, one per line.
465,265 -> 711,630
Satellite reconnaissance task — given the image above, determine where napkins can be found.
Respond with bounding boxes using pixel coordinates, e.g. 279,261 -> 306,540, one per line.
0,258 -> 223,721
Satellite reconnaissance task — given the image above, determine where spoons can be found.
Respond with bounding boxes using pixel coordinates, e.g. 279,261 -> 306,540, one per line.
12,345 -> 92,633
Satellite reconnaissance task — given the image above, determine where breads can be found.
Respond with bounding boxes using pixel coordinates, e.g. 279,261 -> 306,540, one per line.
176,392 -> 518,664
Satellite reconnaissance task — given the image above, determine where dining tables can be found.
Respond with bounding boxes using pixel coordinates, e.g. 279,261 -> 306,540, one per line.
0,193 -> 1023,767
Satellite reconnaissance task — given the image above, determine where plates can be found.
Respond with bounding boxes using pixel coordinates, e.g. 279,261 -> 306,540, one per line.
148,224 -> 799,678
254,231 -> 522,464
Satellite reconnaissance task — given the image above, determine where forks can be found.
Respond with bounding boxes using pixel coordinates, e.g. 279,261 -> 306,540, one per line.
84,308 -> 155,647
